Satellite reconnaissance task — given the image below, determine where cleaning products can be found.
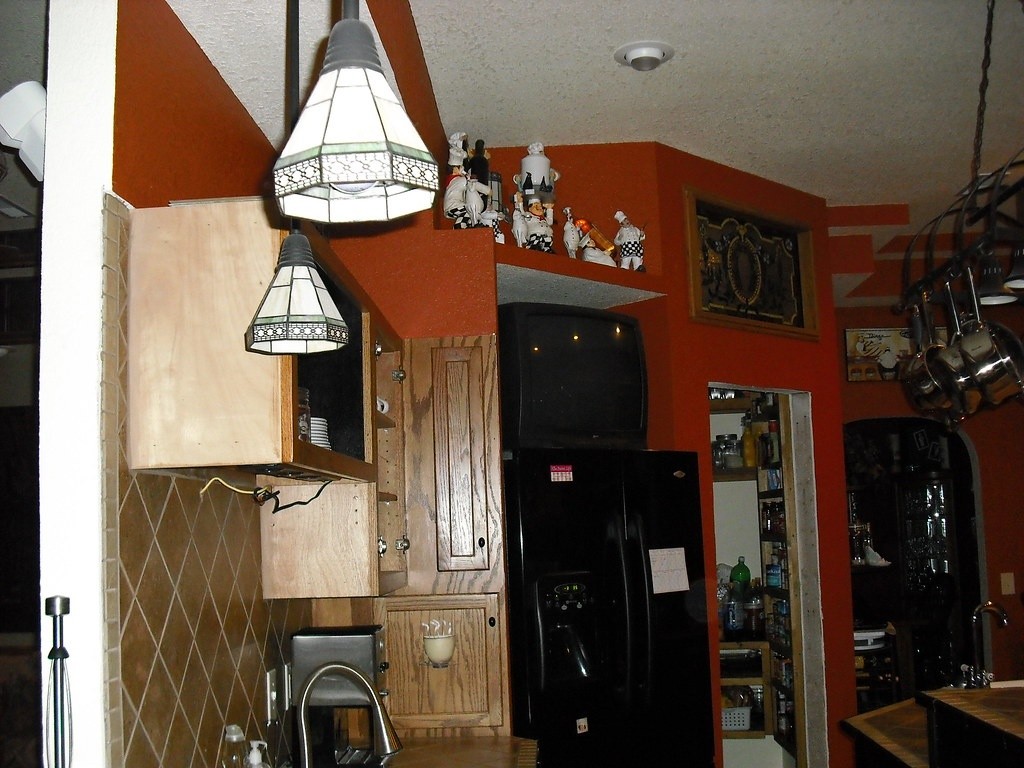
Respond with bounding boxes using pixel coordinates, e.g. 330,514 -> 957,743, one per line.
223,724 -> 272,768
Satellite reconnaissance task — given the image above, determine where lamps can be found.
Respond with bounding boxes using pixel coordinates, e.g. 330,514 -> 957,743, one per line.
272,0 -> 440,225
976,149 -> 1024,304
244,1 -> 350,353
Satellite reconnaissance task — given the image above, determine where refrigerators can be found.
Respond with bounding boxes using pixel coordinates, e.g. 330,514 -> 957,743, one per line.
502,446 -> 718,768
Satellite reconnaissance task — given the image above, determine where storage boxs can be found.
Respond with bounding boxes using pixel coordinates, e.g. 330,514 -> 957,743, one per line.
720,707 -> 751,731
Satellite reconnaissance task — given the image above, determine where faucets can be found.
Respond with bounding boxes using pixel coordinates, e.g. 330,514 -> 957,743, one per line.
295,660 -> 403,768
960,601 -> 1010,688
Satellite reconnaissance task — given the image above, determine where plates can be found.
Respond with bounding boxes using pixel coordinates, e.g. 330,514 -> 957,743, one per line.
309,417 -> 332,451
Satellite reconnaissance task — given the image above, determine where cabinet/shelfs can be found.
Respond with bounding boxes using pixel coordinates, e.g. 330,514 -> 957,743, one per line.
720,641 -> 773,739
709,393 -> 808,768
126,200 -> 409,599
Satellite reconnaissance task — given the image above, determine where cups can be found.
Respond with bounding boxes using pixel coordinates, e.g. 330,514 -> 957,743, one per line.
422,635 -> 456,668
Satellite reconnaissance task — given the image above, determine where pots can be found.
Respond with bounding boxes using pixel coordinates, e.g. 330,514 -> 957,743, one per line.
901,267 -> 1024,427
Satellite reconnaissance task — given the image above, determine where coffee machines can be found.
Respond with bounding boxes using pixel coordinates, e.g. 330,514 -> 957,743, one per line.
289,626 -> 388,767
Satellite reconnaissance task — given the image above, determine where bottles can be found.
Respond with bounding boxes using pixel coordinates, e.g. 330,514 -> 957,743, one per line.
223,724 -> 247,768
712,416 -> 754,470
298,387 -> 311,444
719,556 -> 764,643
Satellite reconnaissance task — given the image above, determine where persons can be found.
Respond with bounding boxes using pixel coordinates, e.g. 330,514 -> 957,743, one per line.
580,231 -> 617,268
444,147 -> 472,230
613,211 -> 646,272
514,191 -> 555,253
